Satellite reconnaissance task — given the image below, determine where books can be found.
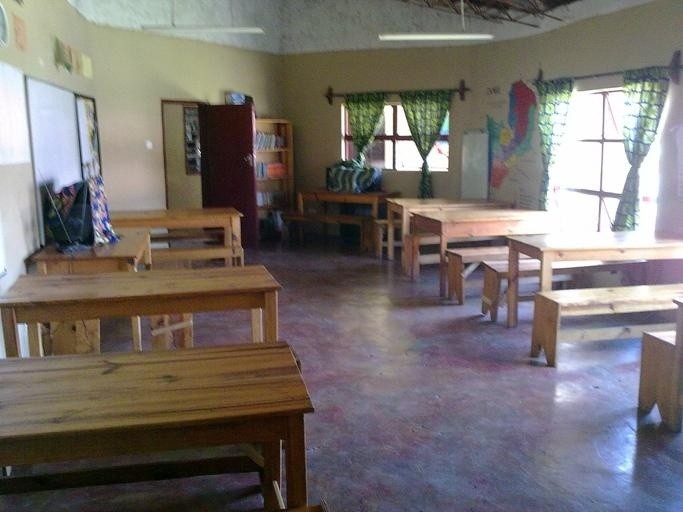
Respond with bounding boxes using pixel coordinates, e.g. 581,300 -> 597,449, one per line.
255,131 -> 287,206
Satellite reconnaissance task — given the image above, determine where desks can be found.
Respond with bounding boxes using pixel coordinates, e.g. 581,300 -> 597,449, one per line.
107,206 -> 246,266
372,187 -> 679,435
2,265 -> 284,360
28,227 -> 153,354
6,340 -> 316,512
294,190 -> 401,251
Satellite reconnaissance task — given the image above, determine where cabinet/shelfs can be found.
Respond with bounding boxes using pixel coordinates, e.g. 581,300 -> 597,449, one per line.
254,119 -> 295,245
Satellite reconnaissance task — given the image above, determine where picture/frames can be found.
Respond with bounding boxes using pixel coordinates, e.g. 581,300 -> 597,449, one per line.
182,106 -> 201,176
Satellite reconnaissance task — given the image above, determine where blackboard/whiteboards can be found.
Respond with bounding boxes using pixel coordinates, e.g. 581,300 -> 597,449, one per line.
26,76 -> 83,247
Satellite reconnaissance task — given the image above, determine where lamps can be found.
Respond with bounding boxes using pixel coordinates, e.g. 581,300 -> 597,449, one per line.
378,0 -> 495,42
142,0 -> 267,38
